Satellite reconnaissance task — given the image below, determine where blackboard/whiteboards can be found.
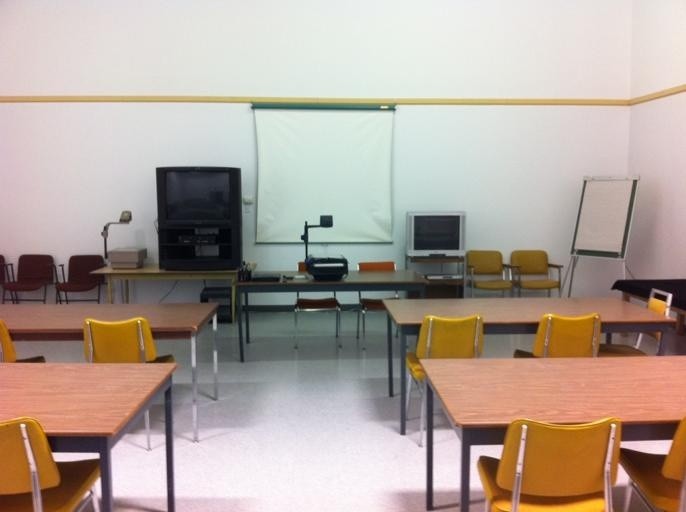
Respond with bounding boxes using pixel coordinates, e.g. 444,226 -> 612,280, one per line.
571,175 -> 640,259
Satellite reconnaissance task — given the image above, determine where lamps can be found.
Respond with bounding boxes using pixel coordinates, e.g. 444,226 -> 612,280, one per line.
101,211 -> 132,266
302,214 -> 333,262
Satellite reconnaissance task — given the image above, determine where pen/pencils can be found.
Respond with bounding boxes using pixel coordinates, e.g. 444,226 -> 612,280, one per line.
238,261 -> 253,272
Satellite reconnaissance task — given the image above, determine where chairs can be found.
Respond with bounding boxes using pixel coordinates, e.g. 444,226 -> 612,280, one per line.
2,255 -> 43,309
293,261 -> 345,349
619,414 -> 686,512
1,319 -> 46,364
81,319 -> 177,453
354,262 -> 407,350
477,417 -> 622,512
511,250 -> 563,299
599,288 -> 673,358
402,314 -> 482,446
512,313 -> 600,359
464,250 -> 514,300
55,254 -> 106,306
0,417 -> 104,512
0,255 -> 13,304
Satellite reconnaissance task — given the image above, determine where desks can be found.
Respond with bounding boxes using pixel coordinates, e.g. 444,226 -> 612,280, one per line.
379,295 -> 668,435
408,256 -> 464,299
418,356 -> 685,511
91,263 -> 240,328
0,362 -> 179,511
0,303 -> 222,440
234,271 -> 428,363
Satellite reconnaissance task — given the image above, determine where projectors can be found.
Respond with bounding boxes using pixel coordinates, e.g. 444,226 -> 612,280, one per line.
102,210 -> 148,270
303,215 -> 349,280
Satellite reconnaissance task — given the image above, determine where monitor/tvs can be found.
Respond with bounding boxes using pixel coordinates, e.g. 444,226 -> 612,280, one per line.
405,210 -> 468,259
154,165 -> 243,227
